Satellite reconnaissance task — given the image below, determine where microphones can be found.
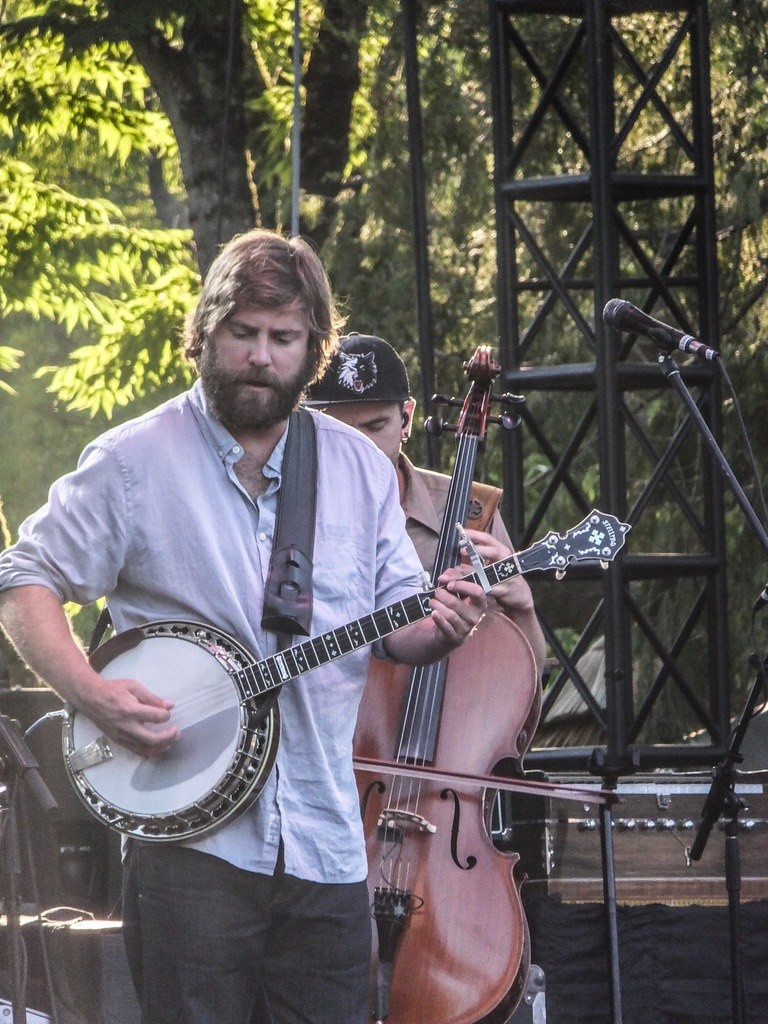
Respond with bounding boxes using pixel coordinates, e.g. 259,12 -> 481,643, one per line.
602,298 -> 720,363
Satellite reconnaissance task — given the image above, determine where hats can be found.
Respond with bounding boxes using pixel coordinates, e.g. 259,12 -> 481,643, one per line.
298,331 -> 411,406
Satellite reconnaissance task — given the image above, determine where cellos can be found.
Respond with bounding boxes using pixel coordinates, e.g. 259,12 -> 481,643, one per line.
350,347 -> 548,1023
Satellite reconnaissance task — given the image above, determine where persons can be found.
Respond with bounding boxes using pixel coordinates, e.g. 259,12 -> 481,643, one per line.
0,228 -> 487,1022
300,333 -> 547,1024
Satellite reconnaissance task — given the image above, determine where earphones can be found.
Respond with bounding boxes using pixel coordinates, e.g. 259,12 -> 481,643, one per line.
402,412 -> 409,428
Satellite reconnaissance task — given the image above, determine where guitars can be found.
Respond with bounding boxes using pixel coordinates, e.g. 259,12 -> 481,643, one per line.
61,509 -> 633,845
